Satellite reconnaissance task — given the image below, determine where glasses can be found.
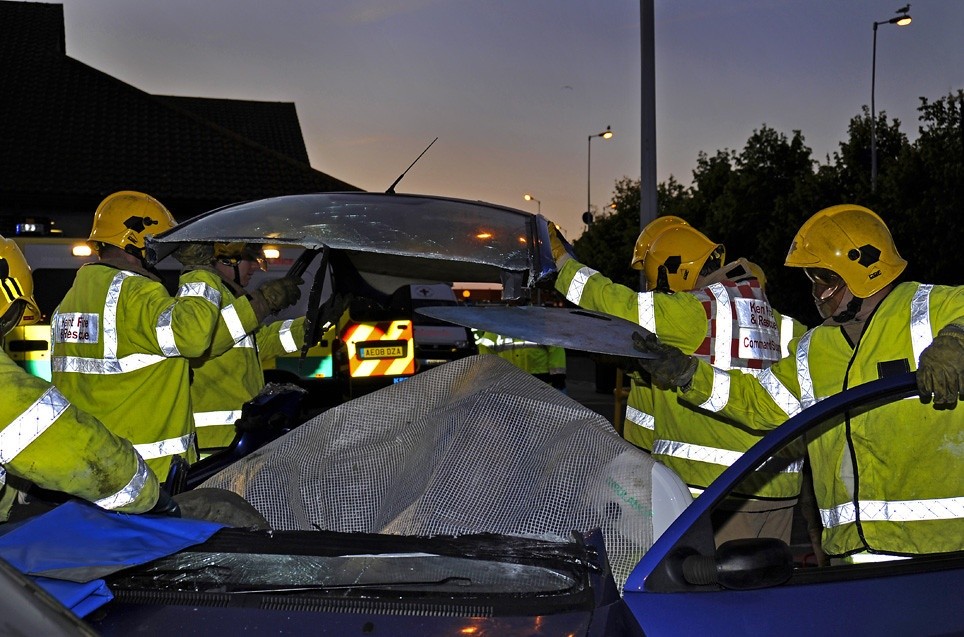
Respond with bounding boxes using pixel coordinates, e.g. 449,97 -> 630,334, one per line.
804,266 -> 837,285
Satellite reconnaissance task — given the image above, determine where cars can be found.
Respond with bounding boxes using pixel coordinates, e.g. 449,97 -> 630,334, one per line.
0,186 -> 964,635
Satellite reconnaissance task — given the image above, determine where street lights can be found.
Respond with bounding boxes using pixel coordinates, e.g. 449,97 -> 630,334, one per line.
587,130 -> 613,232
555,225 -> 568,239
870,14 -> 912,194
523,194 -> 541,215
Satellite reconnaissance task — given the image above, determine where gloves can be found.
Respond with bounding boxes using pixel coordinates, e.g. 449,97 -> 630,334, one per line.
172,240 -> 216,268
261,276 -> 306,313
916,327 -> 964,404
304,287 -> 351,344
630,329 -> 700,390
650,266 -> 675,295
547,219 -> 569,270
150,487 -> 184,519
552,374 -> 568,395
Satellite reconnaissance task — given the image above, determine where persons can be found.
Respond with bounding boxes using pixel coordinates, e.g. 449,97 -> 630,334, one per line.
183,241 -> 322,461
475,214 -> 808,549
633,204 -> 964,567
0,235 -> 180,520
49,191 -> 223,483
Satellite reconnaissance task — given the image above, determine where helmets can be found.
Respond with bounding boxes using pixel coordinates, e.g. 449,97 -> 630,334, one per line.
86,189 -> 180,260
631,215 -> 727,294
214,239 -> 267,265
0,235 -> 42,331
785,204 -> 907,297
724,256 -> 767,295
